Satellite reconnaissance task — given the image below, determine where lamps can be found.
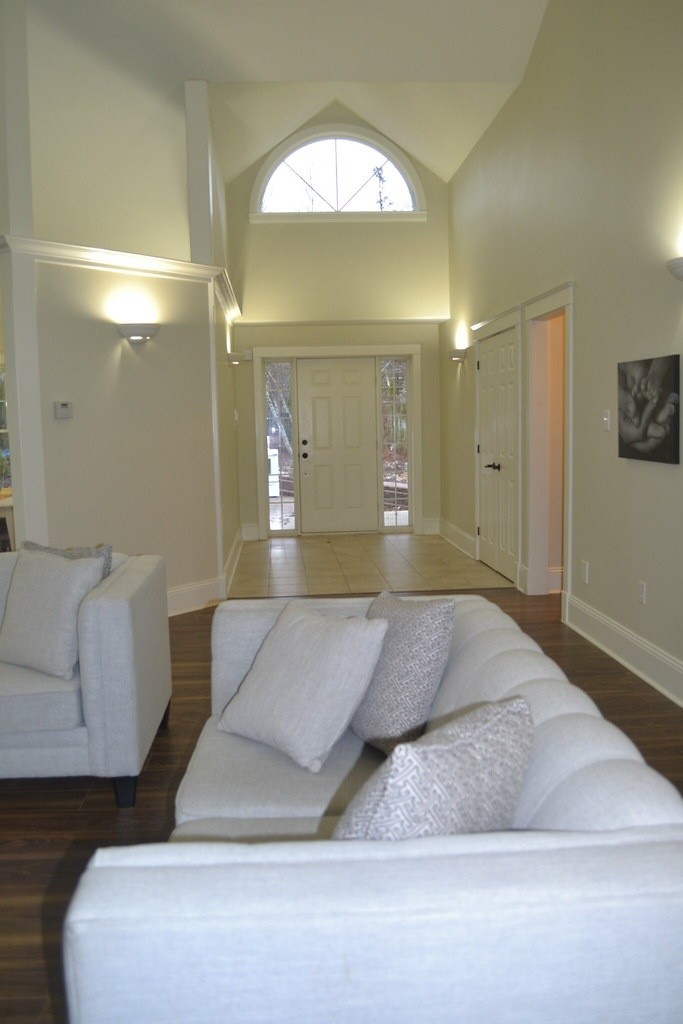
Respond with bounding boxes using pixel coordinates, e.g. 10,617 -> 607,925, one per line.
228,353 -> 243,367
116,323 -> 161,345
450,349 -> 466,362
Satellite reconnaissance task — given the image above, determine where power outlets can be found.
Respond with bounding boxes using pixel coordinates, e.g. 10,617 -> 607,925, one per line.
55,401 -> 73,419
602,409 -> 609,430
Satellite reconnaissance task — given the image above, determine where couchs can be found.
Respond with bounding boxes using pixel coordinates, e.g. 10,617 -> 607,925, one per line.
63,594 -> 683,1024
0,550 -> 172,808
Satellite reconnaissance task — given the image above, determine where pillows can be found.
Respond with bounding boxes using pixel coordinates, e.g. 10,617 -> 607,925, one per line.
0,540 -> 113,679
331,697 -> 534,841
216,599 -> 389,775
349,587 -> 455,757
